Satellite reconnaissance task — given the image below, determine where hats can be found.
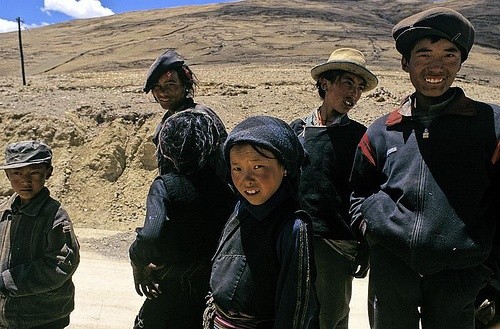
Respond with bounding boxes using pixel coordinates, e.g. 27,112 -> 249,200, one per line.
1,141 -> 53,169
392,6 -> 476,64
309,48 -> 379,95
143,50 -> 185,95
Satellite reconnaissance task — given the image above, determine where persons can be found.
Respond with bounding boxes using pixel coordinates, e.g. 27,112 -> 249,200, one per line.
0,140 -> 80,329
143,49 -> 228,175
348,7 -> 500,329
129,107 -> 238,329
286,47 -> 378,329
202,115 -> 319,329
